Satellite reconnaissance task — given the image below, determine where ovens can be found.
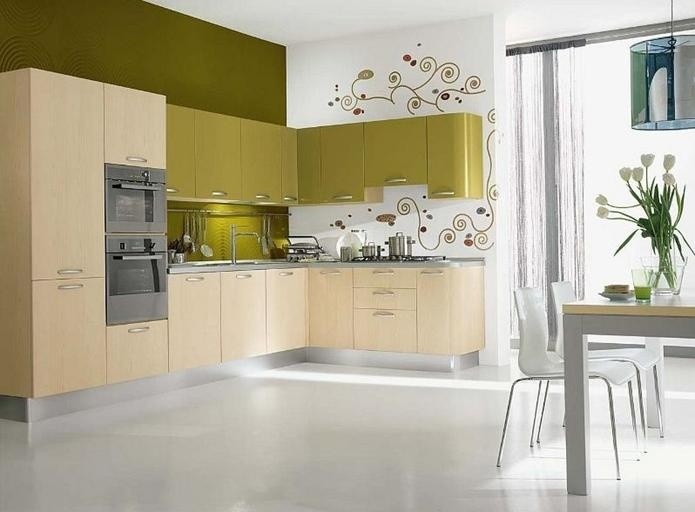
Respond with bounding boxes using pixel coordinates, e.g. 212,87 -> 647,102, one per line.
107,163 -> 167,326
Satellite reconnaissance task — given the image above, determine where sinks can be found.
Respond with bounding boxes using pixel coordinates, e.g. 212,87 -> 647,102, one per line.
191,260 -> 288,267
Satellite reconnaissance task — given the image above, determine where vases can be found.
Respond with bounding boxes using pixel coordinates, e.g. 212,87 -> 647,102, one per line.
640,255 -> 687,295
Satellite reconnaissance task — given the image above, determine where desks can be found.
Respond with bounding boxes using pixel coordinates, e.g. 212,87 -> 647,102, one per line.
562,290 -> 695,495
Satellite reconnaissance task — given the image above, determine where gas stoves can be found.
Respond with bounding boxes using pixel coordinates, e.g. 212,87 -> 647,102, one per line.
355,252 -> 444,262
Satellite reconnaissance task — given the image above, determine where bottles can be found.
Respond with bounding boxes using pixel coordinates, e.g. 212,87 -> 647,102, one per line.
340,246 -> 352,262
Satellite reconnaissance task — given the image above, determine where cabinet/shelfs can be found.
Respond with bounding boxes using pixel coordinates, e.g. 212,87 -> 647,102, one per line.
323,123 -> 364,204
106,320 -> 168,386
365,114 -> 427,204
309,268 -> 354,349
104,82 -> 166,167
353,267 -> 417,353
221,270 -> 267,363
0,69 -> 105,398
426,112 -> 483,199
266,269 -> 306,354
168,273 -> 221,372
195,109 -> 241,202
166,103 -> 195,201
417,267 -> 485,357
281,126 -> 298,204
241,118 -> 282,205
295,126 -> 321,205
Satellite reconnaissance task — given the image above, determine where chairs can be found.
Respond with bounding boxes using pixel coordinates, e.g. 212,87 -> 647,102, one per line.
537,281 -> 665,453
496,287 -> 640,481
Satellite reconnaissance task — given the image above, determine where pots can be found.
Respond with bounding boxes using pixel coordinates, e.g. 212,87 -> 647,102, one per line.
360,241 -> 385,260
385,232 -> 416,256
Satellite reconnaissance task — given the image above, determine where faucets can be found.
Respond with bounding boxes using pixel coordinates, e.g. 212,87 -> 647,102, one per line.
230,223 -> 260,264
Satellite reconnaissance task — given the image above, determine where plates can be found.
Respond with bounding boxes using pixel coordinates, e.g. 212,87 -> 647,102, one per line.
335,233 -> 361,260
599,291 -> 635,300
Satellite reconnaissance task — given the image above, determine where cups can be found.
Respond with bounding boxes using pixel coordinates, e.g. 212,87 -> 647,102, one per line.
629,268 -> 654,305
168,249 -> 185,263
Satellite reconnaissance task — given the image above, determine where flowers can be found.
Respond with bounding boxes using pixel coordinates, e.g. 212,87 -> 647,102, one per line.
596,155 -> 695,290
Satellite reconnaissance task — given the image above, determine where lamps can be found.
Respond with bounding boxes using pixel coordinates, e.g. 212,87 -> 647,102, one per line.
630,0 -> 694,130
